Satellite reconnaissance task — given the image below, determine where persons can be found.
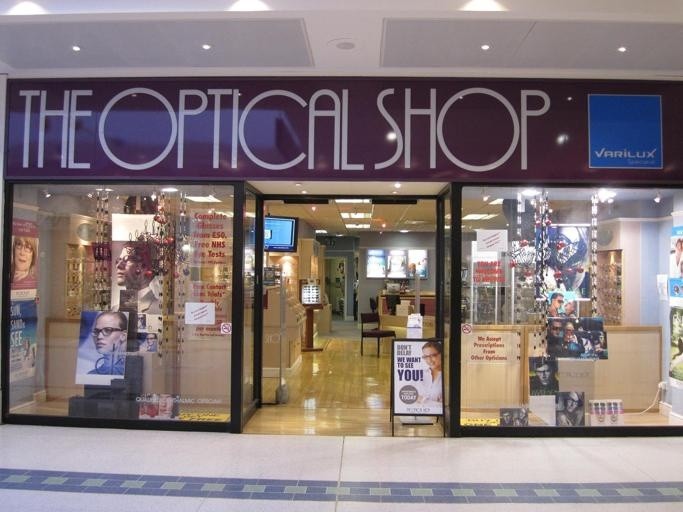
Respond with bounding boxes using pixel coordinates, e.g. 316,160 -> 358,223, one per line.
532,362 -> 559,395
670,239 -> 683,278
556,391 -> 584,426
112,241 -> 163,314
548,293 -> 607,352
422,342 -> 442,403
88,312 -> 128,374
146,333 -> 157,352
11,236 -> 38,290
501,407 -> 529,427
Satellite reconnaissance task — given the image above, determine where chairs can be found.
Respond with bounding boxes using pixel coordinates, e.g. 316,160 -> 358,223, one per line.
360,312 -> 397,358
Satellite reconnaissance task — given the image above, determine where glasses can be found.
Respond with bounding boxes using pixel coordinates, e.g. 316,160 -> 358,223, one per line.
422,350 -> 441,359
535,370 -> 553,376
137,317 -> 145,321
565,397 -> 581,407
146,337 -> 154,340
558,300 -> 564,304
115,256 -> 136,264
552,325 -> 562,331
89,327 -> 124,336
13,240 -> 35,253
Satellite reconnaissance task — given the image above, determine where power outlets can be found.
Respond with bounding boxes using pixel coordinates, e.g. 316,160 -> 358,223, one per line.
659,381 -> 666,390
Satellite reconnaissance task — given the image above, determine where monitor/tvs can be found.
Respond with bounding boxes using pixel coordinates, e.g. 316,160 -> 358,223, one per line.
387,283 -> 400,293
250,216 -> 299,252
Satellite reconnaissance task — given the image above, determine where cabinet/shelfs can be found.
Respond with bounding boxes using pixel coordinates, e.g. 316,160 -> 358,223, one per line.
46,213 -> 108,314
263,283 -> 307,377
